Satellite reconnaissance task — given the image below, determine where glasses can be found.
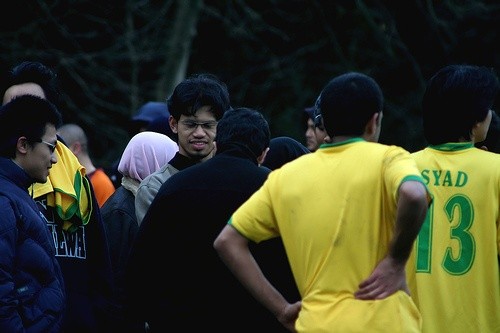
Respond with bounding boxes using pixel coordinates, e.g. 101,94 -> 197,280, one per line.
25,136 -> 57,153
178,119 -> 219,130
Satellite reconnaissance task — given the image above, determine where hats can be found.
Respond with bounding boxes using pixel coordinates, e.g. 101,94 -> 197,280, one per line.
314,95 -> 322,128
132,101 -> 171,129
305,107 -> 314,119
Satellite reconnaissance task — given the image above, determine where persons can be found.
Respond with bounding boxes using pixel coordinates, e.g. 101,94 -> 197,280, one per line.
214,72 -> 432,333
0,59 -> 500,333
